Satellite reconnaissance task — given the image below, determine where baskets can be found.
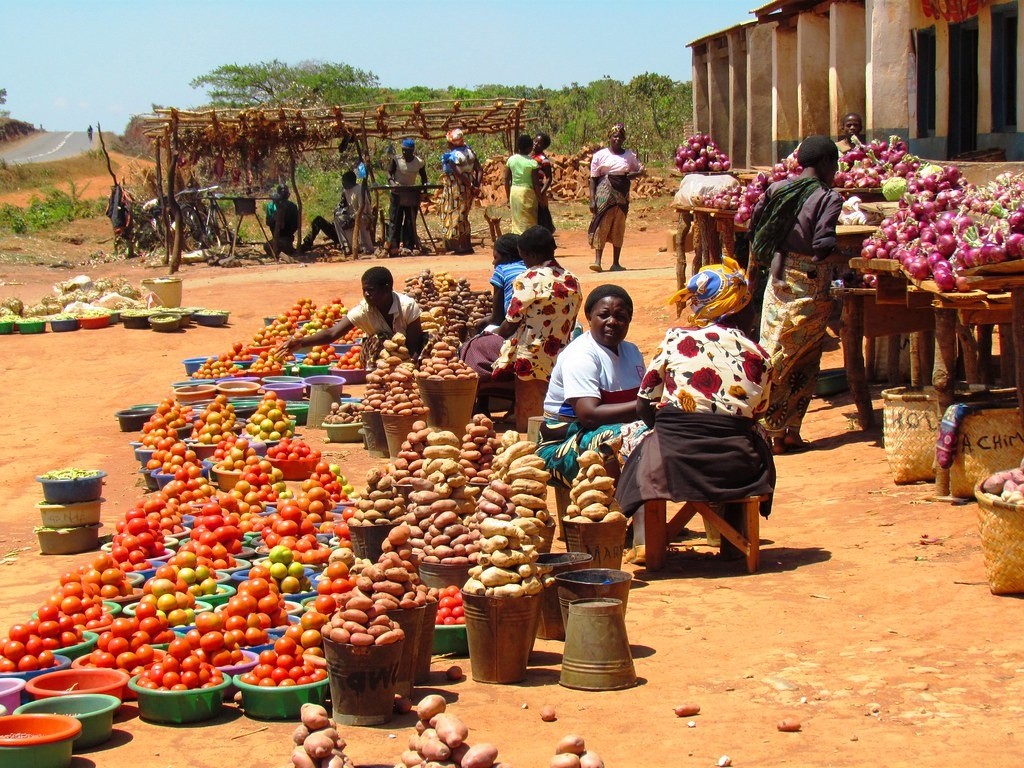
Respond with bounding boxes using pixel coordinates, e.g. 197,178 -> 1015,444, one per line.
879,383 -> 1002,486
950,407 -> 1024,498
975,474 -> 1024,594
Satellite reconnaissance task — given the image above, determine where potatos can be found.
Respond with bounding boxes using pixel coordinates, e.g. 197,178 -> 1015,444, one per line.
981,456 -> 1024,506
292,413 -> 803,768
322,268 -> 494,423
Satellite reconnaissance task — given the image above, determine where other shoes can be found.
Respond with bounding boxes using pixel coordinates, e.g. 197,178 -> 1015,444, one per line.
590,262 -> 602,270
624,543 -> 646,563
609,265 -> 626,271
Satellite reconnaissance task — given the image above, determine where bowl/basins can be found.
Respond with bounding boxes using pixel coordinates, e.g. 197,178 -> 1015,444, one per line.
432,624 -> 469,657
261,376 -> 309,432
79,312 -> 121,329
215,377 -> 265,423
130,423 -> 322,492
298,320 -> 310,326
50,319 -> 78,332
172,376 -> 217,419
36,472 -> 106,555
101,498 -> 357,551
116,403 -> 160,433
264,317 -> 277,326
183,357 -> 218,376
0,548 -> 332,768
122,307 -> 193,330
330,338 -> 372,384
0,321 -> 46,334
284,354 -> 335,378
302,375 -> 368,449
190,308 -> 231,327
230,340 -> 283,371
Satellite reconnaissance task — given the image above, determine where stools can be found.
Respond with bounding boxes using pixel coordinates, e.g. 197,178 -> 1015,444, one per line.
516,377 -> 549,432
552,459 -> 769,575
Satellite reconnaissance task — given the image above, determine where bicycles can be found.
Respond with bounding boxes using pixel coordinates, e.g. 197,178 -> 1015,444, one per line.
145,180 -> 233,259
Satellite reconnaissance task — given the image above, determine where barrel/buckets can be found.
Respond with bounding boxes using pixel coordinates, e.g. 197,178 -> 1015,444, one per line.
321,481 -> 638,726
360,377 -> 479,461
141,278 -> 182,308
307,384 -> 342,429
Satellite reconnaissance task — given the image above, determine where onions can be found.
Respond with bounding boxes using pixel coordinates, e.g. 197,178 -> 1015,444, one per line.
704,140 -> 1024,291
675,134 -> 730,172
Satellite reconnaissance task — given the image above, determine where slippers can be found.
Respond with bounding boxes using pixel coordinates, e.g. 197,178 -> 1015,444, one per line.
788,439 -> 814,453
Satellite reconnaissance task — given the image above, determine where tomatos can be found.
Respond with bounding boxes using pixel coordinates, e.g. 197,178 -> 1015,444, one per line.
0,391 -> 465,688
191,297 -> 364,379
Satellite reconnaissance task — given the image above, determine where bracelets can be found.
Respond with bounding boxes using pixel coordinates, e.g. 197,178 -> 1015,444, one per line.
388,178 -> 394,181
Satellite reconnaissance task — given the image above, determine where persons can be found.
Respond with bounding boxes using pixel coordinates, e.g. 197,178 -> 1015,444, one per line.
615,256 -> 777,566
750,135 -> 841,456
504,132 -> 557,234
303,171 -> 372,251
265,184 -> 298,250
271,266 -> 423,372
461,225 -> 583,425
87,125 -> 93,137
835,113 -> 865,158
388,138 -> 427,251
535,285 -> 647,545
440,128 -> 483,254
588,124 -> 643,271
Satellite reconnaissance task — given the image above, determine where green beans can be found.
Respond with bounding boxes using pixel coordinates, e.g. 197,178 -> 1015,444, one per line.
0,307 -> 226,323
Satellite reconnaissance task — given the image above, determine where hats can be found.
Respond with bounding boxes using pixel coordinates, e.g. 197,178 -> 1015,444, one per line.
668,256 -> 756,329
402,139 -> 416,148
446,129 -> 466,146
608,125 -> 627,141
